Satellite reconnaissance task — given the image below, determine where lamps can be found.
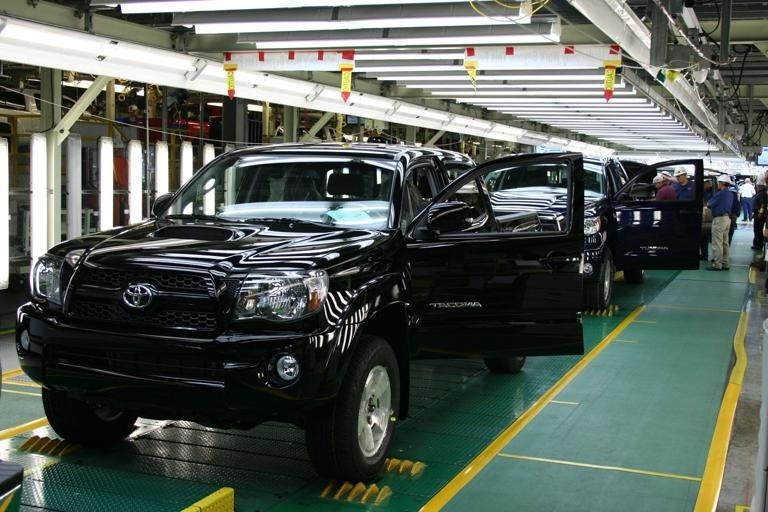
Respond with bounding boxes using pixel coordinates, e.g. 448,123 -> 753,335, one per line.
0,0 -> 724,159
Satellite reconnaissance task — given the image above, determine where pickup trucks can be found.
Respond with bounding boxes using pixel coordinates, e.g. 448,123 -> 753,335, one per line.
135,104 -> 223,143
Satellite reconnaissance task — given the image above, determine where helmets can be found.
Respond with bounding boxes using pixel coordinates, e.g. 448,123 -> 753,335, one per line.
653,167 -> 687,184
717,175 -> 731,184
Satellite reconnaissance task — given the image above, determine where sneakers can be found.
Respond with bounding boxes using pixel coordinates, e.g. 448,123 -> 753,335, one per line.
700,253 -> 729,270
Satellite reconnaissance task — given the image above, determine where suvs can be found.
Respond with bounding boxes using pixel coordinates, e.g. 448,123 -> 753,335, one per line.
621,159 -> 718,257
13,141 -> 587,486
483,145 -> 705,309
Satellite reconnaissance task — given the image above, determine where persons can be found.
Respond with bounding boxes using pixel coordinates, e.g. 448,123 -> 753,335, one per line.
649,166 -> 768,263
704,174 -> 734,272
